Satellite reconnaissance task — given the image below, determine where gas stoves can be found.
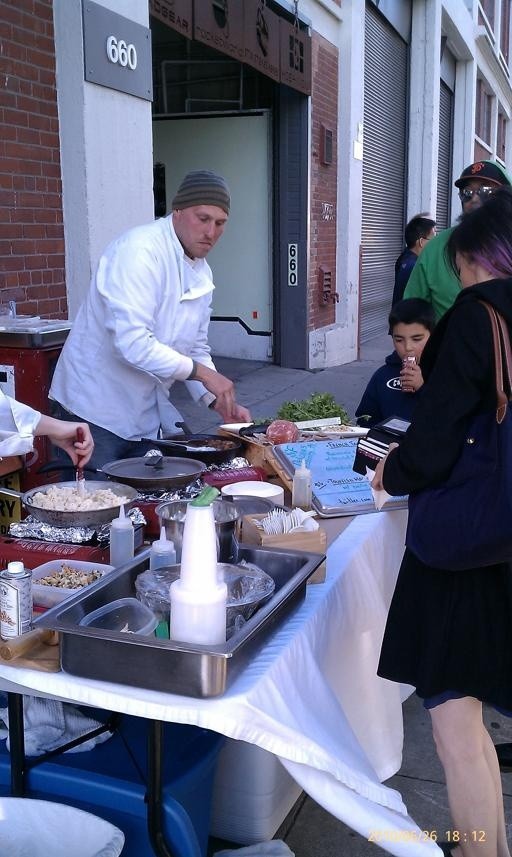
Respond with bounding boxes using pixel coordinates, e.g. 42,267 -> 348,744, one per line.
1,519 -> 144,578
199,461 -> 267,491
130,485 -> 212,533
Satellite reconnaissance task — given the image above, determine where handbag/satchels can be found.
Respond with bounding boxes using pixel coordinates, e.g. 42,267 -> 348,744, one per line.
403,391 -> 512,573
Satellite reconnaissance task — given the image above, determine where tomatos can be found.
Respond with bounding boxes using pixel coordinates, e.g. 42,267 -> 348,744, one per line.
265,420 -> 298,444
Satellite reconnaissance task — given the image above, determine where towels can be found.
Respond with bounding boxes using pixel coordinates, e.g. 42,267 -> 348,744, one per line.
0,687 -> 115,758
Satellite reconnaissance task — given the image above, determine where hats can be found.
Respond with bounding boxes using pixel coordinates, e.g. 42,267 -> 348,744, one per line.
172,170 -> 231,218
454,159 -> 512,188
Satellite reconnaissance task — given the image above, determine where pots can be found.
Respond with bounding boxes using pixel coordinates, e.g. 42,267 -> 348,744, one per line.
36,452 -> 208,488
154,499 -> 245,562
1,480 -> 139,526
162,422 -> 242,462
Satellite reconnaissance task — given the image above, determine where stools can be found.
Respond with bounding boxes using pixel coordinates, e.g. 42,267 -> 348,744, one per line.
0,790 -> 127,856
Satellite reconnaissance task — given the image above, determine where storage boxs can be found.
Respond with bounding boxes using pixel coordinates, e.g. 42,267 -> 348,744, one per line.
213,731 -> 307,847
0,695 -> 218,856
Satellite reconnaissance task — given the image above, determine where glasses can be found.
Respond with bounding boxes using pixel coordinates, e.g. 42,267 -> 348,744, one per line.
457,186 -> 497,203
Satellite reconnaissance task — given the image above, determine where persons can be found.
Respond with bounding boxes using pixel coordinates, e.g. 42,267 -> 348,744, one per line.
387,217 -> 437,337
48,169 -> 251,485
369,204 -> 512,857
0,389 -> 94,467
355,298 -> 437,430
403,159 -> 512,324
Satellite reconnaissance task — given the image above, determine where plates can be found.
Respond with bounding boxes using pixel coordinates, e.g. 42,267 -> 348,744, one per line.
221,481 -> 285,506
218,422 -> 372,435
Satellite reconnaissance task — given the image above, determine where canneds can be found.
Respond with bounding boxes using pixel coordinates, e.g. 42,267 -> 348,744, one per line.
401,354 -> 418,393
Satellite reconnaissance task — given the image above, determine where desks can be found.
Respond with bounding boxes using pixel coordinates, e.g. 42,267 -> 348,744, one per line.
0,472 -> 451,856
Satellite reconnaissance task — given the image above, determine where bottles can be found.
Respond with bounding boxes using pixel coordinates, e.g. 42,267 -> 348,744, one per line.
1,560 -> 34,642
149,526 -> 178,568
402,349 -> 419,392
107,503 -> 135,567
292,458 -> 313,510
170,502 -> 228,646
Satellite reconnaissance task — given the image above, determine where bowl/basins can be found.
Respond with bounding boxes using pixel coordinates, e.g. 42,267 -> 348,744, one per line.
133,562 -> 276,630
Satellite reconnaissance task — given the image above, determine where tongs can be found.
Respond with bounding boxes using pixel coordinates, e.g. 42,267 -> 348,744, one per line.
139,436 -> 220,452
75,425 -> 86,495
